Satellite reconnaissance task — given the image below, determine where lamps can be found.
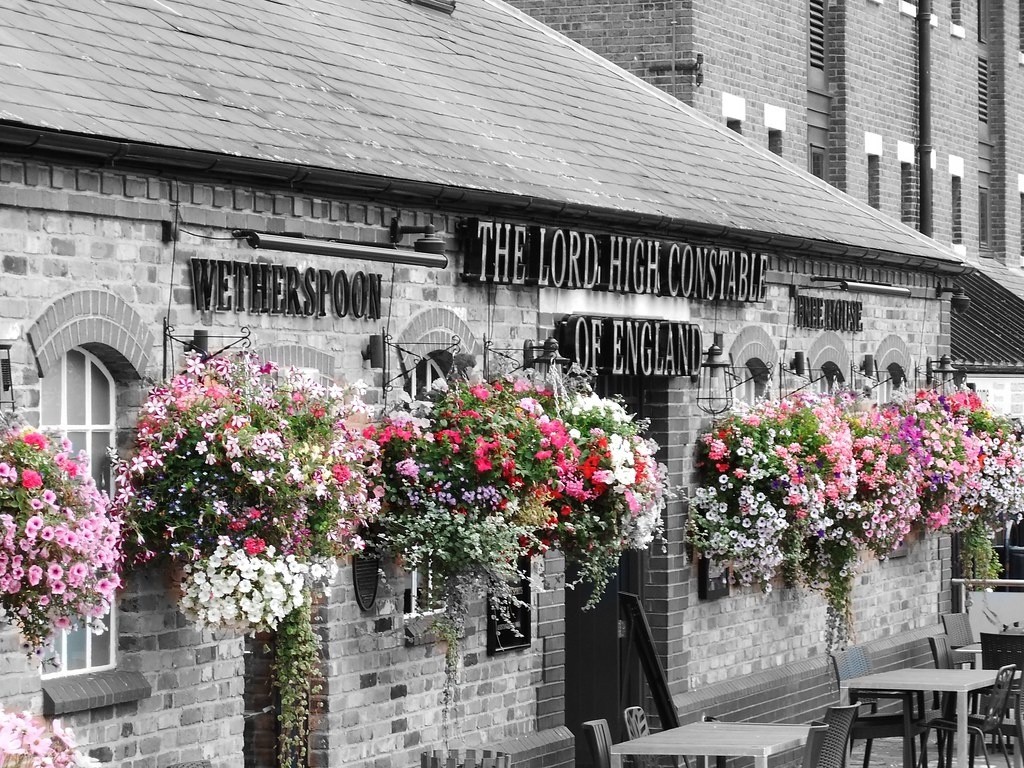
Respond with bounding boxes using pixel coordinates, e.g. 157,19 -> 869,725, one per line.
694,337 -> 737,418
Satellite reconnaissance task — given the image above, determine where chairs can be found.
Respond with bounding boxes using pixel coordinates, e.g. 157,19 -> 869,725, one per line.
928,635 -> 982,738
625,705 -> 663,768
583,717 -> 618,768
941,610 -> 976,649
827,645 -> 928,768
980,631 -> 1024,673
913,662 -> 1017,767
801,719 -> 831,768
817,700 -> 863,768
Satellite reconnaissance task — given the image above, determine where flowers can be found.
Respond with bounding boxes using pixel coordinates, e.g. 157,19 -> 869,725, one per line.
0,354 -> 670,664
689,365 -> 1022,592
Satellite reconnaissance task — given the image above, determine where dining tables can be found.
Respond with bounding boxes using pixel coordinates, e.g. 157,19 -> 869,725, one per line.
608,719 -> 814,768
838,664 -> 1021,768
